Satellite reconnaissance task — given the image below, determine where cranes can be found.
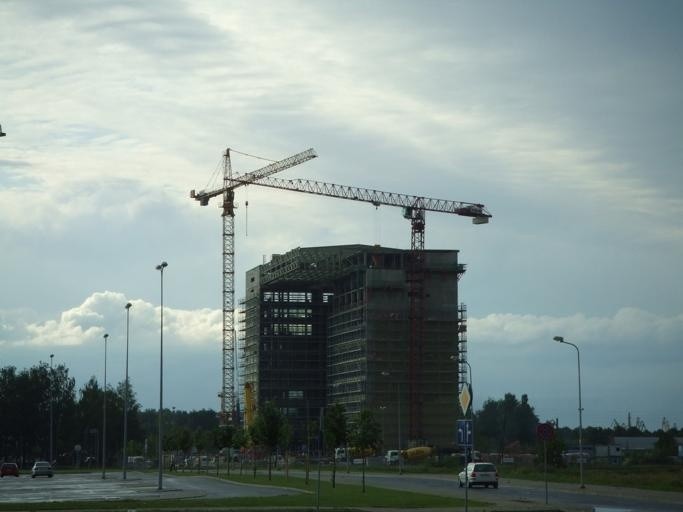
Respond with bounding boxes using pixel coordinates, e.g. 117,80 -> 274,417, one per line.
188,147 -> 319,434
222,171 -> 493,448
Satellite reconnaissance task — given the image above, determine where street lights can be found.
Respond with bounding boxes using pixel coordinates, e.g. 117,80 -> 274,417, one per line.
553,335 -> 587,490
381,370 -> 404,477
49,352 -> 55,464
451,355 -> 475,464
122,301 -> 133,478
101,331 -> 109,481
155,260 -> 168,489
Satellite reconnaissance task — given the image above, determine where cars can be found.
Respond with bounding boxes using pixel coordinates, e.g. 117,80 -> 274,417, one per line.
192,447 -> 286,466
457,461 -> 499,488
31,461 -> 54,478
0,462 -> 20,477
451,450 -> 515,464
